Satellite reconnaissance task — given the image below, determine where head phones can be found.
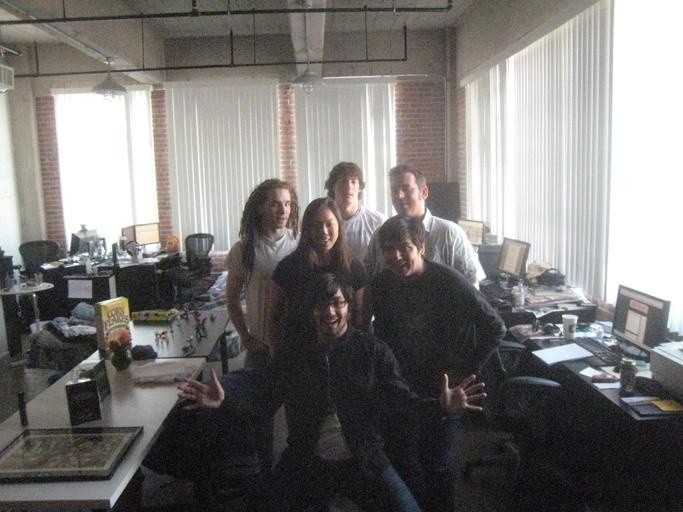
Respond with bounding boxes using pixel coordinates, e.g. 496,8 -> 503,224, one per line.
532,318 -> 561,337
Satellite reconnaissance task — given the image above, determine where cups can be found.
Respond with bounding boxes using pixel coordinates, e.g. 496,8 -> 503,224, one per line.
512,286 -> 529,307
34,273 -> 44,286
562,314 -> 577,342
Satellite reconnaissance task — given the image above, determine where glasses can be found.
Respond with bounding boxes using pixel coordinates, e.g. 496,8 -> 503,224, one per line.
315,299 -> 347,309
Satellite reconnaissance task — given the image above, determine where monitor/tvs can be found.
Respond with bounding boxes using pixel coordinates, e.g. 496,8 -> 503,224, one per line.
497,237 -> 532,291
612,285 -> 671,360
457,219 -> 485,250
70,229 -> 97,259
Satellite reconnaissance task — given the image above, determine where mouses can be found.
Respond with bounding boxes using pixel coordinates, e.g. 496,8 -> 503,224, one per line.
590,374 -> 616,384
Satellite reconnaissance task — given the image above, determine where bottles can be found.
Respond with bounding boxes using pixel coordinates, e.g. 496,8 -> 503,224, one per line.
620,359 -> 637,395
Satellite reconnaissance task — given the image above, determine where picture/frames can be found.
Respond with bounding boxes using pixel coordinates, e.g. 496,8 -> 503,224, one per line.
0,426 -> 144,483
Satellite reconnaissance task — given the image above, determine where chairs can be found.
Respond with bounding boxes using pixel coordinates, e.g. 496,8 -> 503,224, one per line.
19,240 -> 62,297
161,233 -> 215,308
475,340 -> 564,441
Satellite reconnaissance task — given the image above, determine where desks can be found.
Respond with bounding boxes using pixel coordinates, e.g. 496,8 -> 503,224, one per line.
0,357 -> 206,511
0,283 -> 54,326
51,249 -> 180,307
508,320 -> 682,453
481,272 -> 598,325
87,307 -> 232,374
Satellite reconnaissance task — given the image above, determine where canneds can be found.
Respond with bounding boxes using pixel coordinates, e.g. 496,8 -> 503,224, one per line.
619,357 -> 637,398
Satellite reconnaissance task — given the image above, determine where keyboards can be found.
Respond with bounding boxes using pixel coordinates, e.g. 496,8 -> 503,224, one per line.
560,336 -> 621,369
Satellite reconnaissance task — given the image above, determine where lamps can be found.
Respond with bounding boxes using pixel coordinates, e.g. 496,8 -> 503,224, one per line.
292,0 -> 324,94
93,51 -> 128,99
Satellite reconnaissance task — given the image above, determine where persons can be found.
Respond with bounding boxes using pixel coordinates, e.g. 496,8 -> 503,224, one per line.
270,198 -> 366,508
369,215 -> 508,511
364,166 -> 486,291
178,270 -> 486,512
324,163 -> 375,338
223,178 -> 304,464
